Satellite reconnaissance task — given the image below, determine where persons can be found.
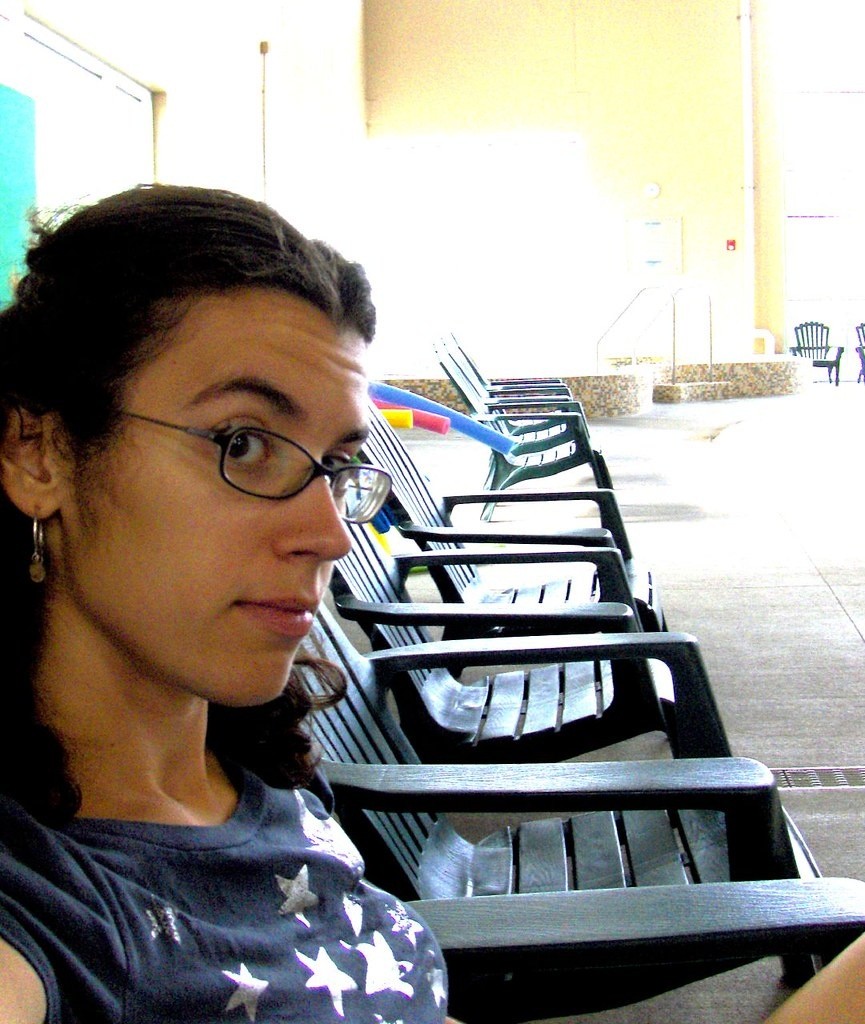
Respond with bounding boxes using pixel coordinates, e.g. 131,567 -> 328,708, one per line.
0,183 -> 865,1024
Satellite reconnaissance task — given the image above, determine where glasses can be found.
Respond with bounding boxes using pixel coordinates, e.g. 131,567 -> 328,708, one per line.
109,407 -> 393,523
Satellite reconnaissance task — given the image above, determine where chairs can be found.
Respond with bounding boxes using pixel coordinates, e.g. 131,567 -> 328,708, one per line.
298,317 -> 865,1024
